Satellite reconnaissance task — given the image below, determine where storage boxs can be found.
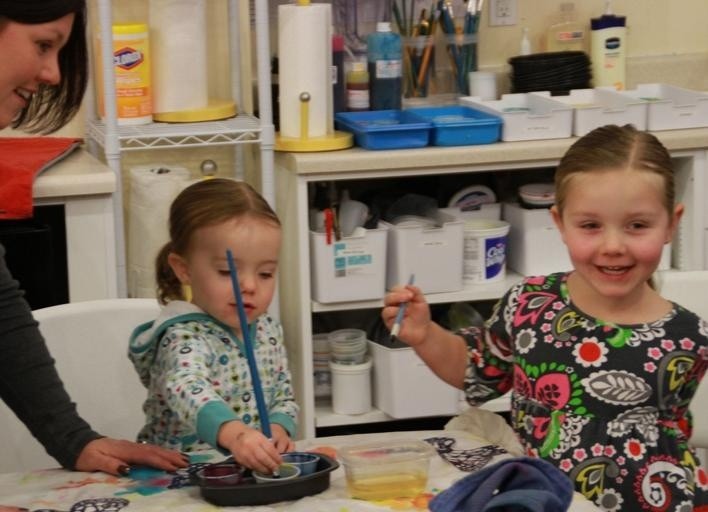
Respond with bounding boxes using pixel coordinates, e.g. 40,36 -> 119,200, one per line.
333,109 -> 431,148
404,106 -> 505,145
531,88 -> 649,136
610,84 -> 707,133
367,339 -> 512,419
309,198 -> 671,302
457,93 -> 575,141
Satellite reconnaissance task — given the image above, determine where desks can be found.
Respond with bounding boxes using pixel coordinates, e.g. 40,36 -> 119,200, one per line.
1,429 -> 602,511
1,152 -> 118,308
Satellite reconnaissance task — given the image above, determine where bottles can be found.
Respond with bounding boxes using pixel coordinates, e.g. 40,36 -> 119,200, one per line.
346,21 -> 403,111
90,0 -> 154,126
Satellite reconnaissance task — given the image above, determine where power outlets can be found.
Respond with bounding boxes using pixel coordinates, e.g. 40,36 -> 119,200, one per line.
488,1 -> 519,27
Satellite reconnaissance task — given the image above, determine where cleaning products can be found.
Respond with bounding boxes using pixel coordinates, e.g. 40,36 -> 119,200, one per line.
589,0 -> 629,90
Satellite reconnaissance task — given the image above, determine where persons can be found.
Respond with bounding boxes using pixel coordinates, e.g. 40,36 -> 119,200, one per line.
128,177 -> 299,476
0,0 -> 190,476
380,124 -> 708,512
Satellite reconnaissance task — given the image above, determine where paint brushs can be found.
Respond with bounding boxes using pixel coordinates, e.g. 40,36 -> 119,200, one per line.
392,1 -> 483,98
227,250 -> 282,479
386,278 -> 412,345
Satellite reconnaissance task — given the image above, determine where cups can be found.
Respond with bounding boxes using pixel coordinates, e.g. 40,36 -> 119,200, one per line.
327,328 -> 375,415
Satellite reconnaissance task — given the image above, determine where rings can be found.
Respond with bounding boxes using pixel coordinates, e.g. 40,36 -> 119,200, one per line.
138,441 -> 148,449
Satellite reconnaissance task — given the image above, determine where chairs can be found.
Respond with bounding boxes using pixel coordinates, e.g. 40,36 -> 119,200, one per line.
660,271 -> 707,470
1,297 -> 164,470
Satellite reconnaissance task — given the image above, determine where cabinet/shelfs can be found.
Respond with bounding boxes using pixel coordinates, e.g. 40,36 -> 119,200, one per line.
275,128 -> 708,439
85,1 -> 273,298
